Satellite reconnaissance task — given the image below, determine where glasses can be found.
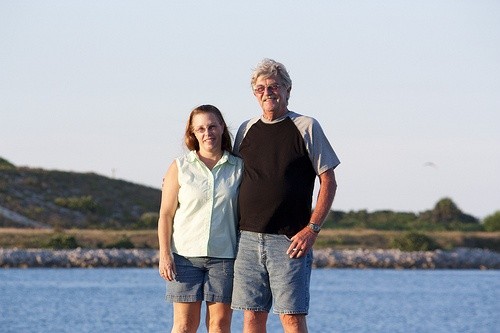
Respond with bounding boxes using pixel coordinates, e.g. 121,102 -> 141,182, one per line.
193,123 -> 221,133
255,84 -> 283,93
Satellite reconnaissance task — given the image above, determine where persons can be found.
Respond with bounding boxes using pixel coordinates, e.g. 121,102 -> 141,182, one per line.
161,58 -> 337,333
158,105 -> 244,333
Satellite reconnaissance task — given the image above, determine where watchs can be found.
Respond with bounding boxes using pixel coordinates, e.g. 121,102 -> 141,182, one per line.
307,223 -> 321,232
